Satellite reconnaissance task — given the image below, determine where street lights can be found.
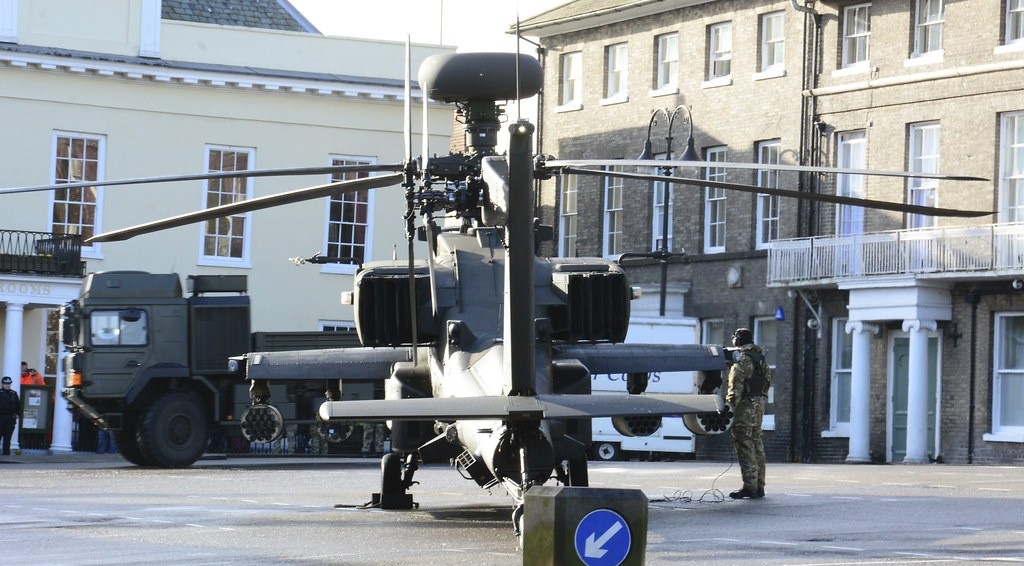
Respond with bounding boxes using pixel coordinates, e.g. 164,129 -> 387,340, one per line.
637,105 -> 704,317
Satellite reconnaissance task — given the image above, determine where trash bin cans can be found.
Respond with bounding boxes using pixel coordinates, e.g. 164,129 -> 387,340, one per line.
21,385 -> 50,433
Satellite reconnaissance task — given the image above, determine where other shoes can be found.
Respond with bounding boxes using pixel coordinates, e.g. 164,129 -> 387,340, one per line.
757,486 -> 765,497
729,488 -> 758,499
3,450 -> 10,455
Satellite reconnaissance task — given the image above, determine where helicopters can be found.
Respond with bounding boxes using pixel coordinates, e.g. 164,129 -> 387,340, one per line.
0,16 -> 1000,535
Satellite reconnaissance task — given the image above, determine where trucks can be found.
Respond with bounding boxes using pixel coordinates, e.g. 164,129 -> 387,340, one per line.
577,316 -> 701,461
58,271 -> 386,468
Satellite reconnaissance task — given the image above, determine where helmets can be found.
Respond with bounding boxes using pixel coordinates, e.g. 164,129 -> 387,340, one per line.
1,376 -> 12,384
735,328 -> 753,341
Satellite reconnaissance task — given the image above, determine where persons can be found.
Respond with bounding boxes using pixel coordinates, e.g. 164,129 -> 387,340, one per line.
723,327 -> 773,500
0,377 -> 22,455
21,362 -> 46,385
